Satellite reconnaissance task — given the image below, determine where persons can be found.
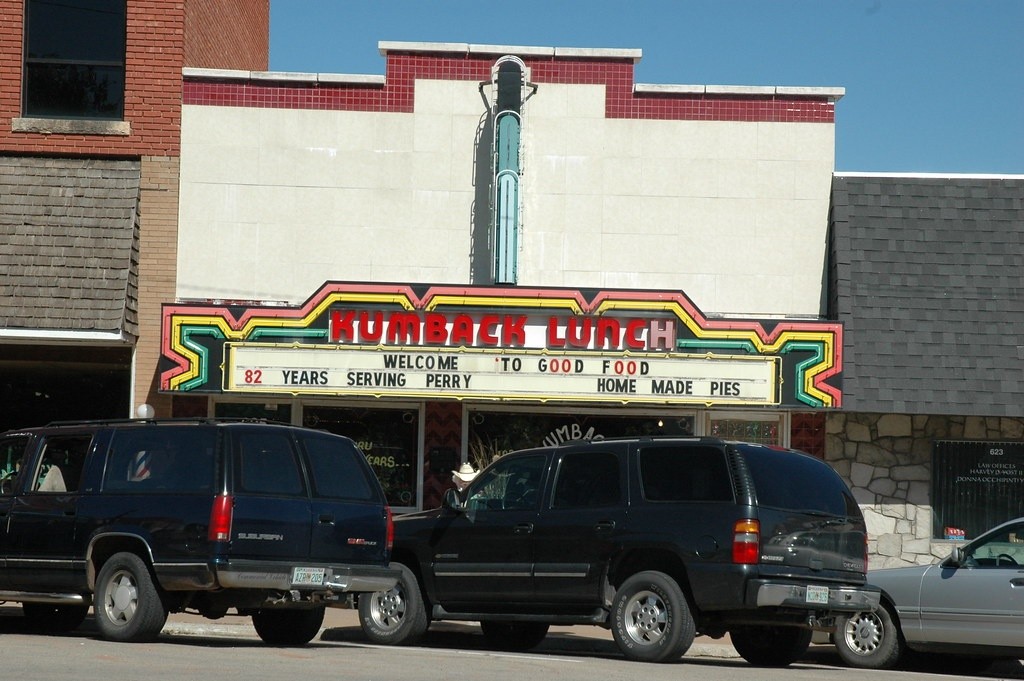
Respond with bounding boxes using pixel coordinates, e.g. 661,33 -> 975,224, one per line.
451,461 -> 482,506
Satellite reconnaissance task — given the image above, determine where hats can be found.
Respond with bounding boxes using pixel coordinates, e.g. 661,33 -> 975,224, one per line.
451,462 -> 480,482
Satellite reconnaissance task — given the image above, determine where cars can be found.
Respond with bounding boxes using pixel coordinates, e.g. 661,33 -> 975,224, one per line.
809,515 -> 1024,669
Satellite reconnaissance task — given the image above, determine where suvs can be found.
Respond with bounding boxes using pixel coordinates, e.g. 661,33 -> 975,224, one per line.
358,433 -> 884,667
0,416 -> 405,647
